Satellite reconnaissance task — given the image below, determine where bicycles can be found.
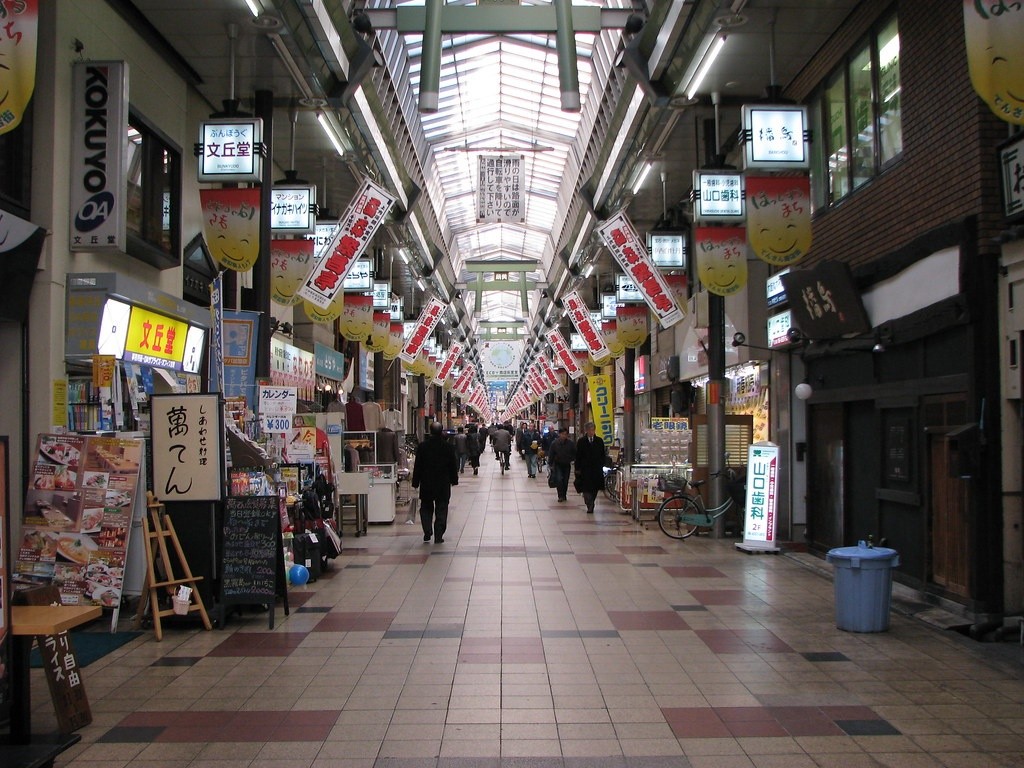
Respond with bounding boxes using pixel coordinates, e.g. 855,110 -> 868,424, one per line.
537,454 -> 546,474
657,453 -> 748,539
498,451 -> 506,475
603,452 -> 624,503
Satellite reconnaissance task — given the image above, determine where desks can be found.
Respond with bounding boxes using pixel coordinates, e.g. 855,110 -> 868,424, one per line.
10,603 -> 104,768
335,472 -> 370,537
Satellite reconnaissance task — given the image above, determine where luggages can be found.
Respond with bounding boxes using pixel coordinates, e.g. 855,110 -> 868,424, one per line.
294,533 -> 321,582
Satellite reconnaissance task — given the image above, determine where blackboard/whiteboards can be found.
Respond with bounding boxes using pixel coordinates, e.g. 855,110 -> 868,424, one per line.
220,495 -> 288,606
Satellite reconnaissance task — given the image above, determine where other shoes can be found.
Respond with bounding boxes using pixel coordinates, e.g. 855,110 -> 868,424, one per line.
457,470 -> 464,473
505,467 -> 509,469
558,496 -> 566,501
528,474 -> 535,478
587,506 -> 593,513
474,468 -> 478,475
496,457 -> 499,460
424,531 -> 445,543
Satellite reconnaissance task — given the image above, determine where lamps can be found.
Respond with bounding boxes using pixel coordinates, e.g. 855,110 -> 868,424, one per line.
337,384 -> 344,395
415,279 -> 426,292
585,264 -> 596,279
385,225 -> 400,245
279,322 -> 295,338
795,374 -> 826,401
447,329 -> 453,336
316,382 -> 332,392
872,332 -> 894,353
683,27 -> 733,100
312,108 -> 347,157
544,310 -> 567,347
239,0 -> 266,19
397,248 -> 412,265
408,264 -> 419,279
650,108 -> 686,157
731,332 -> 805,355
265,32 -> 315,100
633,160 -> 656,196
591,246 -> 605,263
729,0 -> 750,16
345,159 -> 366,189
441,318 -> 447,325
787,323 -> 895,345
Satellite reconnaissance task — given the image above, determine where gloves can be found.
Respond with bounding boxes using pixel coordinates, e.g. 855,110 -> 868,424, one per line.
522,450 -> 525,454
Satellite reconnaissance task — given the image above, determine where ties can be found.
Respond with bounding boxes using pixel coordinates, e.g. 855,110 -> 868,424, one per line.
590,438 -> 592,443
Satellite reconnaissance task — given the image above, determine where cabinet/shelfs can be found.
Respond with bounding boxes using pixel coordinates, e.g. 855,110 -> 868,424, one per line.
368,482 -> 396,525
67,375 -> 123,434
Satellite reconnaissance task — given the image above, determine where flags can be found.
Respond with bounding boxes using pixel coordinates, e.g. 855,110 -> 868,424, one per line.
432,341 -> 464,387
545,327 -> 583,380
500,352 -> 562,422
296,179 -> 397,310
597,211 -> 685,329
561,290 -> 611,361
397,296 -> 447,364
586,375 -> 614,446
451,362 -> 493,416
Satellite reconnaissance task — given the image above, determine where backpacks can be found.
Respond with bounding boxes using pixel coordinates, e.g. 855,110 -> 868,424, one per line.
304,491 -> 321,519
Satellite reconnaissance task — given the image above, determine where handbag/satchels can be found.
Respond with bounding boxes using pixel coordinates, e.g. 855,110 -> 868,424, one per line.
294,518 -> 337,533
548,475 -> 555,488
477,442 -> 483,453
312,474 -> 335,518
323,519 -> 343,559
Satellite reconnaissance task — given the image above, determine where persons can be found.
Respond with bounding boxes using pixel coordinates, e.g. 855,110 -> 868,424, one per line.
412,422 -> 459,545
574,422 -> 606,514
454,421 -> 559,476
294,416 -> 305,426
547,427 -> 577,502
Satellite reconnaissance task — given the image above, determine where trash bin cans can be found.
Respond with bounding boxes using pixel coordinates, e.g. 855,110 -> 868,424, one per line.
826,538 -> 899,635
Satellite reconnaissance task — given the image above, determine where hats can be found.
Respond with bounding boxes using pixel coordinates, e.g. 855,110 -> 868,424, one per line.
548,425 -> 554,431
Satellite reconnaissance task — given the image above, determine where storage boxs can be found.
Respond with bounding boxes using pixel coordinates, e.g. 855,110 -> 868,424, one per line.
357,461 -> 398,483
